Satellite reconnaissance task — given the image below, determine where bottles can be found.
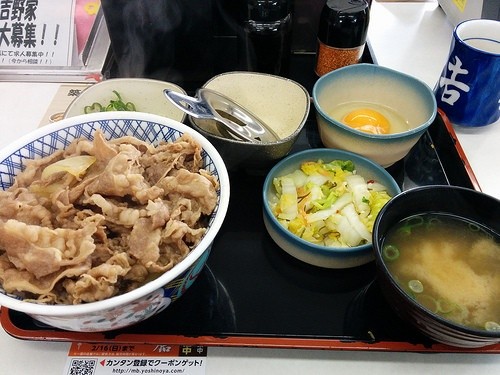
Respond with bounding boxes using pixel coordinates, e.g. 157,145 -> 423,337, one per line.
313,0 -> 370,83
237,1 -> 294,76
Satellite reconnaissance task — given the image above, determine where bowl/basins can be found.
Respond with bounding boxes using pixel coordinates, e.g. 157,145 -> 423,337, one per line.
372,184 -> 500,348
64,77 -> 188,124
189,70 -> 310,164
263,147 -> 400,268
312,63 -> 438,168
0,111 -> 230,331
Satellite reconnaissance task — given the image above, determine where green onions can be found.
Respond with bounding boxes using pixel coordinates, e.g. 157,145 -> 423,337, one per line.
383,216 -> 500,333
84,90 -> 138,114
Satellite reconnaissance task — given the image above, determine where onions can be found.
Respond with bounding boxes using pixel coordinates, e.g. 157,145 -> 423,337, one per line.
40,155 -> 95,180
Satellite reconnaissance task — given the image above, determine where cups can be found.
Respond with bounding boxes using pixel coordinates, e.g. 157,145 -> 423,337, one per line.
431,18 -> 500,128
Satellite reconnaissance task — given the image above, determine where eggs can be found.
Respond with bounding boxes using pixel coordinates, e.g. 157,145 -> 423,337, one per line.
329,100 -> 410,135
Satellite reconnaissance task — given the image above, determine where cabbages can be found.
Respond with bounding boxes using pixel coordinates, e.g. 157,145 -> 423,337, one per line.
266,160 -> 393,247
